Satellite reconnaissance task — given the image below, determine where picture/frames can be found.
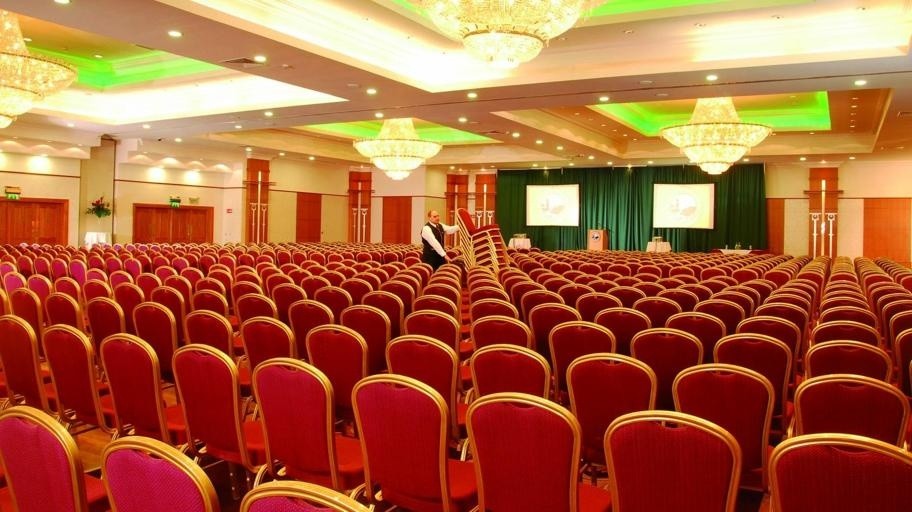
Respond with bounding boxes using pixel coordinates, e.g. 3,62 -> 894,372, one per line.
352,115 -> 446,181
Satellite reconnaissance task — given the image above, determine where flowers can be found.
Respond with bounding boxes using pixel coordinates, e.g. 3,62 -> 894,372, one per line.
87,195 -> 112,217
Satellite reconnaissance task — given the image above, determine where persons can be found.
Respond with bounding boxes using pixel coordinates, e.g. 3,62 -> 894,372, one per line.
421,210 -> 459,270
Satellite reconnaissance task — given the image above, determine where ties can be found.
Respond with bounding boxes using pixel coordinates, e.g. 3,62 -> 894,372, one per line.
436,224 -> 439,229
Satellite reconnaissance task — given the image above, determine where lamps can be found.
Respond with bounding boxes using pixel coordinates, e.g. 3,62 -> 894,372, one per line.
407,0 -> 605,65
659,94 -> 776,178
1,9 -> 79,131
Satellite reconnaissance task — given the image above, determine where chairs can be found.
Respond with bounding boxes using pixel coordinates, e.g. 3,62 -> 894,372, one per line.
351,373 -> 479,512
454,207 -> 512,279
0,311 -> 59,418
465,242 -> 912,439
669,361 -> 777,494
465,390 -> 611,512
565,351 -> 659,489
602,408 -> 744,511
252,356 -> 367,503
100,331 -> 190,457
239,478 -> 375,512
1,402 -> 106,512
384,333 -> 469,463
170,343 -> 269,491
1,239 -> 464,412
783,371 -> 910,449
40,324 -> 118,435
98,433 -> 222,511
767,433 -> 912,511
